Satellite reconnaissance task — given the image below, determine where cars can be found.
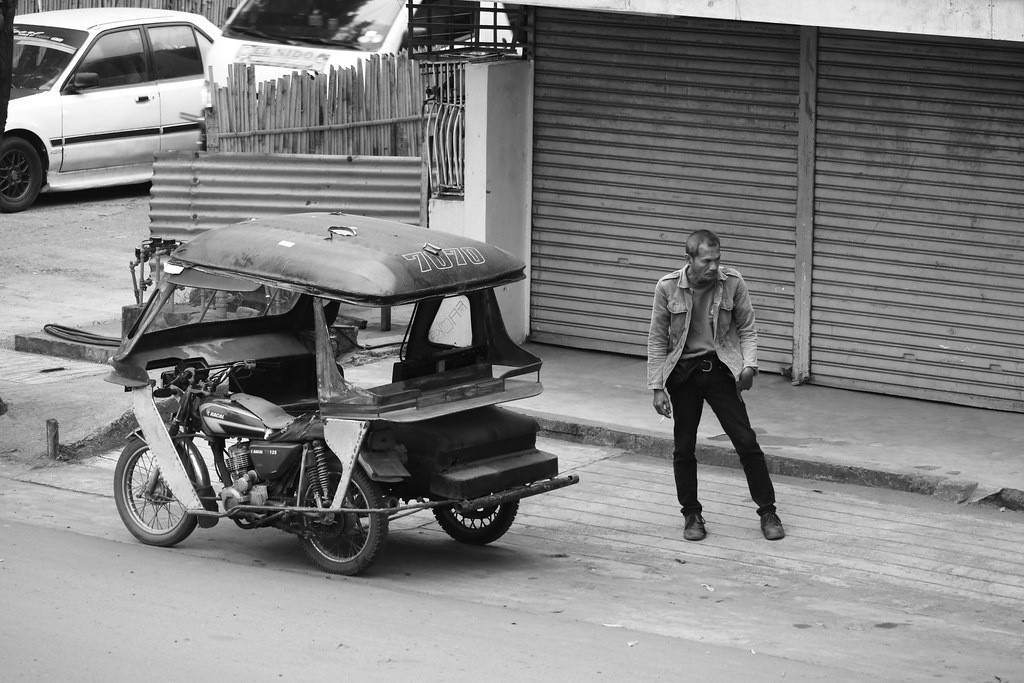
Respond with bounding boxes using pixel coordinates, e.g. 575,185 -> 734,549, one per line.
0,4 -> 224,214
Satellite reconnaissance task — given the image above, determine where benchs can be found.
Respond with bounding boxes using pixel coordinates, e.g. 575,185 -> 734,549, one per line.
391,342 -> 486,383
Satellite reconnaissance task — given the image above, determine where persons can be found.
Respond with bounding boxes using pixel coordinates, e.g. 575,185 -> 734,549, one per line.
647,229 -> 786,541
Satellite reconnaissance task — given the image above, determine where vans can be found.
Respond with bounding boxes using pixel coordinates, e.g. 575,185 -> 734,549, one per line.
205,1 -> 483,97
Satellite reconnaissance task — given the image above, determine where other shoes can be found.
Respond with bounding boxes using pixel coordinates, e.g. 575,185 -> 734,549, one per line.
683,512 -> 706,540
761,512 -> 784,539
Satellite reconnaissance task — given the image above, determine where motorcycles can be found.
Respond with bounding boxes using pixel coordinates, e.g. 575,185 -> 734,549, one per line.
102,206 -> 580,577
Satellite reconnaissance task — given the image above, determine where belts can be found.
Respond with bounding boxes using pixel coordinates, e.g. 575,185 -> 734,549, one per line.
699,359 -> 720,372
